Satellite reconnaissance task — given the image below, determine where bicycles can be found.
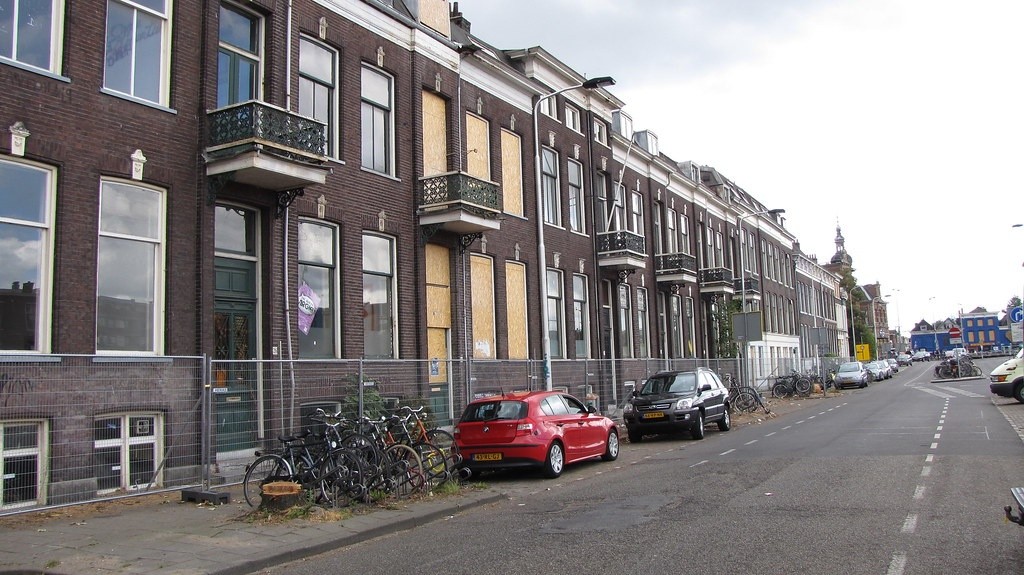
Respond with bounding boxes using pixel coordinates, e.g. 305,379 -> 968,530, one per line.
720,371 -> 761,415
244,405 -> 465,518
772,368 -> 833,398
935,352 -> 982,378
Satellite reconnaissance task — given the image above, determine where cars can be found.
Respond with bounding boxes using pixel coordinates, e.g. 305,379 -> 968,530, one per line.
453,382 -> 619,485
868,351 -> 930,382
833,361 -> 869,389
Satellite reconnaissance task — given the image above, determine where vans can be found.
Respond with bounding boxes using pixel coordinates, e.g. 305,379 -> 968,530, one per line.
989,347 -> 1024,402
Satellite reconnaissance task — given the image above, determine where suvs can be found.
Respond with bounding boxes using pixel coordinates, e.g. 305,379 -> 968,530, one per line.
623,366 -> 734,444
